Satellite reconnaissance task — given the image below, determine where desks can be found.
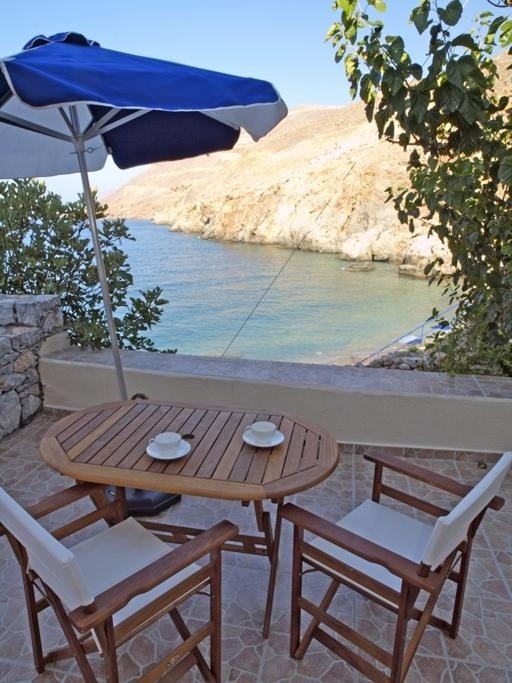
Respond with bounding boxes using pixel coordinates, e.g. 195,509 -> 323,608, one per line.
40,400 -> 338,638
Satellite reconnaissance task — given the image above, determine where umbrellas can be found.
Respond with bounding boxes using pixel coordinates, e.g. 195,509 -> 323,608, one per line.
0,32 -> 287,401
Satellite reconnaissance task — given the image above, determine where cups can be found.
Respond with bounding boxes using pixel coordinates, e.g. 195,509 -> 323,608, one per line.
245,421 -> 276,440
149,432 -> 181,452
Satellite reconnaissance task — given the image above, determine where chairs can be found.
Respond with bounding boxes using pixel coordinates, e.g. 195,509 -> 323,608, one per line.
281,448 -> 512,683
0,482 -> 238,683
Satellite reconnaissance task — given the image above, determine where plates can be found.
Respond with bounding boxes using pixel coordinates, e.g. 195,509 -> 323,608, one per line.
242,431 -> 284,447
145,440 -> 191,460
